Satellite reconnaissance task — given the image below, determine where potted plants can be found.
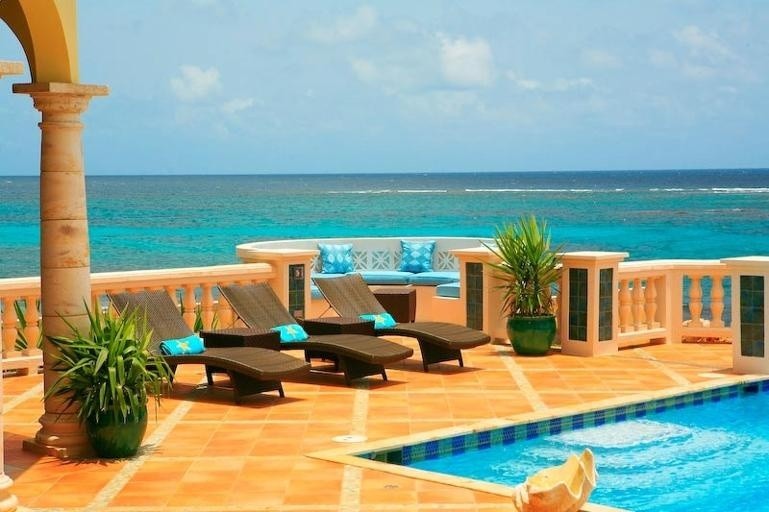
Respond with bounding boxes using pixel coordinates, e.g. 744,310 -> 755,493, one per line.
474,213 -> 569,356
40,295 -> 179,458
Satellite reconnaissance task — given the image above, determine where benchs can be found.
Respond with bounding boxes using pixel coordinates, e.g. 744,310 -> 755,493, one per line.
311,238 -> 461,324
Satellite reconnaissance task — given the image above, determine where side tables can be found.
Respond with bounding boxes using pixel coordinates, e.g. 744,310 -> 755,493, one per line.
373,289 -> 416,323
199,327 -> 281,386
303,317 -> 374,364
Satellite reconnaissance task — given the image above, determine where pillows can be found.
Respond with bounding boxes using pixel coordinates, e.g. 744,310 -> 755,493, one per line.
317,243 -> 356,273
398,240 -> 436,272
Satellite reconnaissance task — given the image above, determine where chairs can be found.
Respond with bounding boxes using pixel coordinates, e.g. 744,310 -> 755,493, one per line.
106,293 -> 312,406
311,273 -> 490,372
217,283 -> 414,387
